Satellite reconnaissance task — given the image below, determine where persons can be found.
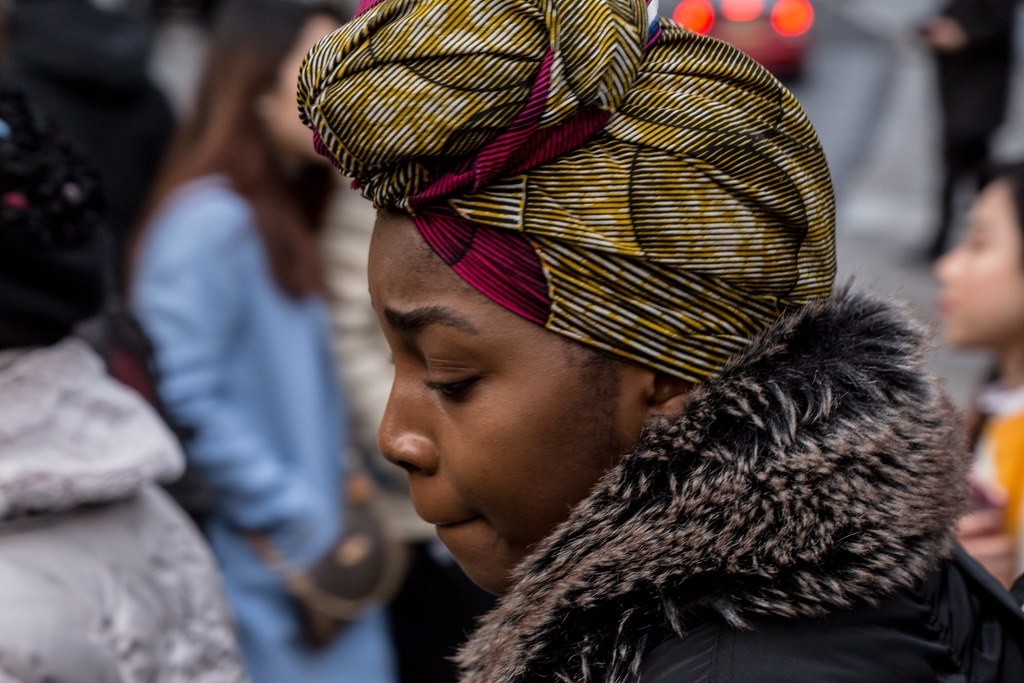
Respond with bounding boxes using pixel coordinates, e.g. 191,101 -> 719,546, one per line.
0,0 -> 501,683
296,0 -> 1024,683
